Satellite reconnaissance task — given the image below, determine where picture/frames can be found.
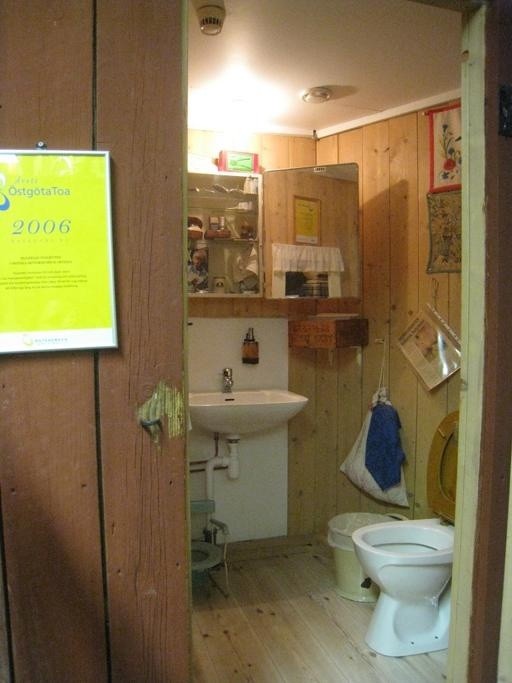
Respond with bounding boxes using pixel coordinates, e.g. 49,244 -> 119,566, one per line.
293,195 -> 322,247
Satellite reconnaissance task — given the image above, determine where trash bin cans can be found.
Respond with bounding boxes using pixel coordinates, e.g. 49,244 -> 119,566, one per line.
327,511 -> 398,603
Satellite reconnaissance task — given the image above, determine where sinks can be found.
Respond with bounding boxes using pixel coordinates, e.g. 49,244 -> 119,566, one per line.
188,389 -> 310,433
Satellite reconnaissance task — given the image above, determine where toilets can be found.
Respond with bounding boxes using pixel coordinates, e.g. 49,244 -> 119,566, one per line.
352,410 -> 460,658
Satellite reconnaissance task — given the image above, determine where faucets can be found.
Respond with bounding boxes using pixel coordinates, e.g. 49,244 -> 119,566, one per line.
222,368 -> 234,393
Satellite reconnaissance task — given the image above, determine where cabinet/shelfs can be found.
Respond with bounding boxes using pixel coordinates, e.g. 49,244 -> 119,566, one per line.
186,170 -> 265,299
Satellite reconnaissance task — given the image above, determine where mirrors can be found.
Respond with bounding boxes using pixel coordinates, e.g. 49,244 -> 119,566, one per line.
264,162 -> 362,299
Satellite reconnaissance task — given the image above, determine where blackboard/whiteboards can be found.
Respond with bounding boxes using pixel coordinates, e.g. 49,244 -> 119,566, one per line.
1,150 -> 119,353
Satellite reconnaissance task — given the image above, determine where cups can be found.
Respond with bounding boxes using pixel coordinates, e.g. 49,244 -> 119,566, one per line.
212,276 -> 226,293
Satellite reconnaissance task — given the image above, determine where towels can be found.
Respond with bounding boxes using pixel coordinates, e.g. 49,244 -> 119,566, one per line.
366,401 -> 406,491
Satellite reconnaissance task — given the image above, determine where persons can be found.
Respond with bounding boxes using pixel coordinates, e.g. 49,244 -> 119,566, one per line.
188,249 -> 209,290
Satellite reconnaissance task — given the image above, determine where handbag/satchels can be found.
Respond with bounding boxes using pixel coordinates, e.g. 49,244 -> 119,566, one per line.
337,388 -> 412,510
